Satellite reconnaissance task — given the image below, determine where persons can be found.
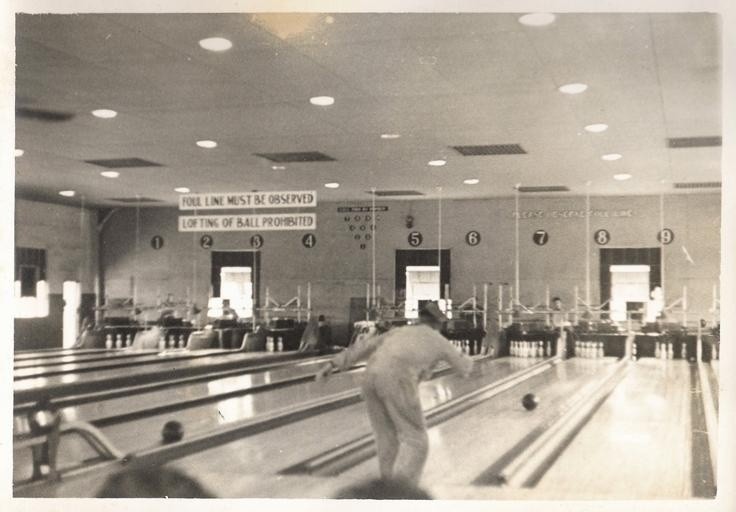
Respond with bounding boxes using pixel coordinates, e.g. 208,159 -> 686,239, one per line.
315,303 -> 473,488
553,297 -> 564,310
